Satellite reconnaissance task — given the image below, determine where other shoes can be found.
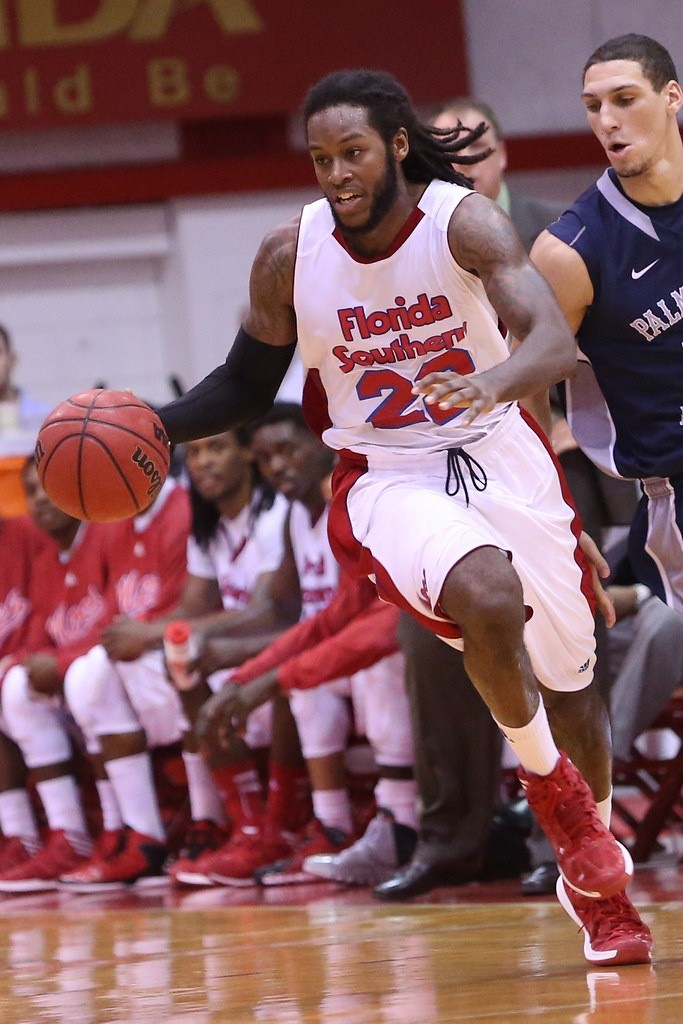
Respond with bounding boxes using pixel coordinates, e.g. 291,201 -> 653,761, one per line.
369,850 -> 467,900
519,860 -> 566,897
304,818 -> 403,887
524,837 -> 560,872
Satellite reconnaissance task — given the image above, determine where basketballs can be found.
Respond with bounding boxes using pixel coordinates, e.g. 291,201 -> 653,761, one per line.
33,387 -> 171,524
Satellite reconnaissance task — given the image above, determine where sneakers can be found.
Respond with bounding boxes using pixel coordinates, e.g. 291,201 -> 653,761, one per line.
134,820 -> 231,888
168,831 -> 256,886
200,825 -> 298,886
253,818 -> 359,887
0,828 -> 96,893
553,874 -> 655,968
517,751 -> 636,898
0,835 -> 42,874
56,823 -> 174,894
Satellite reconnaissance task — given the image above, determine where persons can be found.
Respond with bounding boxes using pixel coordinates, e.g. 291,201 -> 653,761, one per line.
504,34 -> 683,614
148,69 -> 653,965
0,98 -> 683,897
